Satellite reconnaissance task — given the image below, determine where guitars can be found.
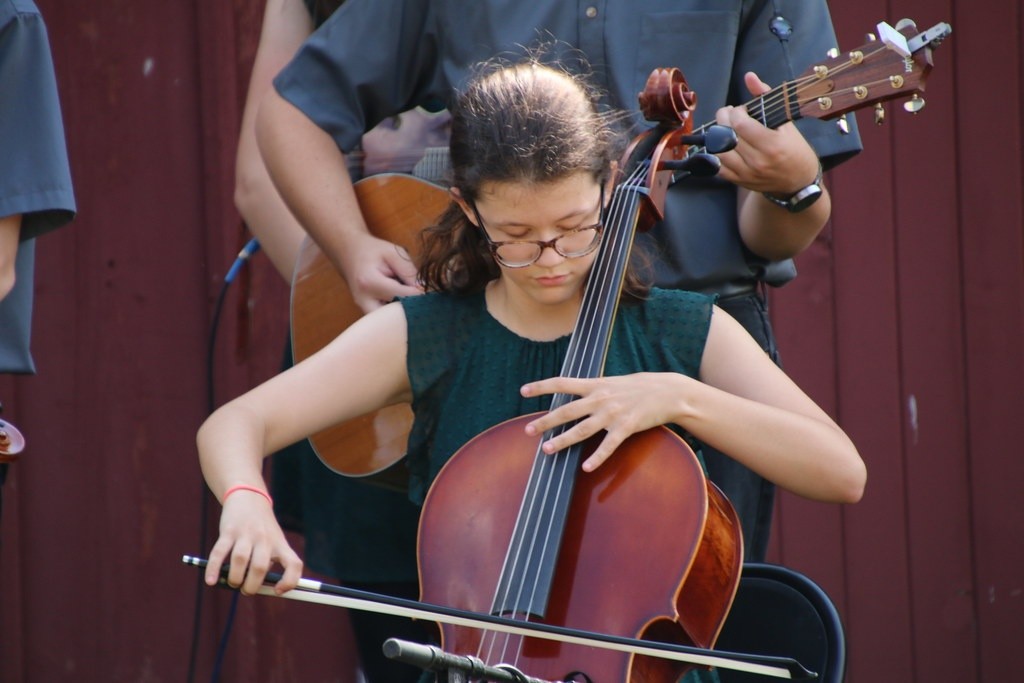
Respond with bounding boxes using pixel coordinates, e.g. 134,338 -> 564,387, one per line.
290,19 -> 953,481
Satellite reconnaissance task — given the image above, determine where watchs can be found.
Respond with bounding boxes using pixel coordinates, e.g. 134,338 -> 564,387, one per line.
761,153 -> 823,214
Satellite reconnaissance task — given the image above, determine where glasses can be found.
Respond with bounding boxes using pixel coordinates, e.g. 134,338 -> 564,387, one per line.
470,181 -> 606,268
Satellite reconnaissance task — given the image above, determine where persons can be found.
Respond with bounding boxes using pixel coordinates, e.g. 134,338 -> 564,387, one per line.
196,56 -> 868,682
2,1 -> 82,377
232,0 -> 869,680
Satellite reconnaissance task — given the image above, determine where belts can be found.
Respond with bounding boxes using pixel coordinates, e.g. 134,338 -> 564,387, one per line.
682,279 -> 759,304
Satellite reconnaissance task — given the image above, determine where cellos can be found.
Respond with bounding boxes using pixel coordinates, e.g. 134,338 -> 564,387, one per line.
412,62 -> 745,683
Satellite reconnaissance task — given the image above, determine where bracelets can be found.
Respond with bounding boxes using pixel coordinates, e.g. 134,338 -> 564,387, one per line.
221,485 -> 275,509
